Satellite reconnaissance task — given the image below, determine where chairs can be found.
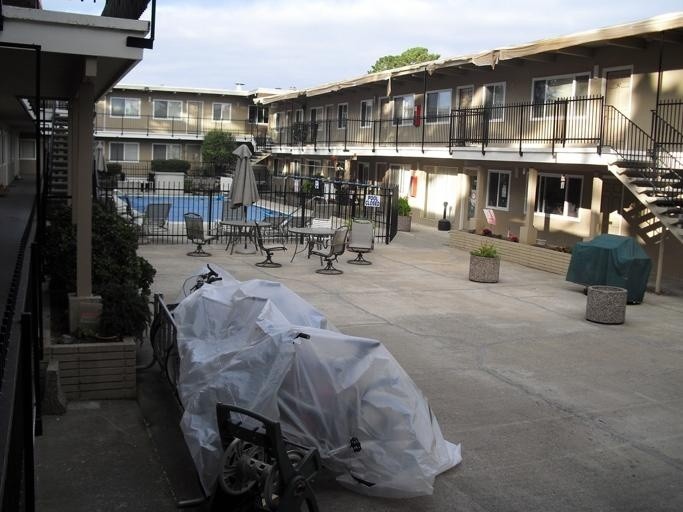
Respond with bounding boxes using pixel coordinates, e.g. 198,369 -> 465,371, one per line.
184,193 -> 376,277
138,203 -> 170,233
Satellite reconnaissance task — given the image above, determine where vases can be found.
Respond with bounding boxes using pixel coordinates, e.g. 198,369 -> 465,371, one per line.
584,284 -> 627,324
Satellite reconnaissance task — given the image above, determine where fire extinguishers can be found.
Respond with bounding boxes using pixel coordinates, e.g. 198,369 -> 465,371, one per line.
414,105 -> 420,127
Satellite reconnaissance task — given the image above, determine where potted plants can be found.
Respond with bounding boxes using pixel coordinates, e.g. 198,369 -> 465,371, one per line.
469,242 -> 501,284
397,198 -> 412,232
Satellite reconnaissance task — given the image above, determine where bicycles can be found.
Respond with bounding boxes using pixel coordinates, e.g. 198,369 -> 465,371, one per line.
150,263 -> 436,488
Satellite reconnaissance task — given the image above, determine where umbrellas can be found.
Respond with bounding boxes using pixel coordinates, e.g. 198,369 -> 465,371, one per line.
97,142 -> 108,177
231,144 -> 259,249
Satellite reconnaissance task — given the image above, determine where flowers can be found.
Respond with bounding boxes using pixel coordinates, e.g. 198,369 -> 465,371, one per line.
481,228 -> 493,236
510,236 -> 518,242
